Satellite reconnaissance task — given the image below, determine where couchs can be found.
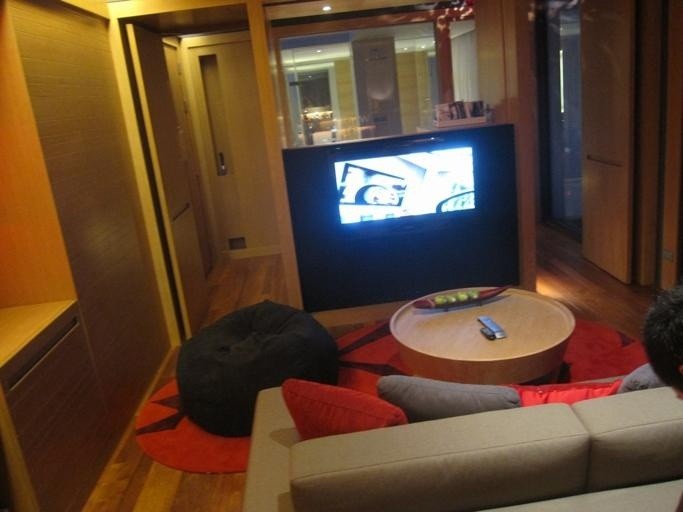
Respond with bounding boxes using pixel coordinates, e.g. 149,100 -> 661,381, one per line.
242,373 -> 683,511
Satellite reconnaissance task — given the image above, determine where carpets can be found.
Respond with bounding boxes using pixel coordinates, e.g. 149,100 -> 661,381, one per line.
132,312 -> 658,474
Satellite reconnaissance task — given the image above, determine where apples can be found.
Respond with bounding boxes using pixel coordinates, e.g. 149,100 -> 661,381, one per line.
435,289 -> 481,306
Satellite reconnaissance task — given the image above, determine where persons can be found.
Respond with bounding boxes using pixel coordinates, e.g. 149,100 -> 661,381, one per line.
639,285 -> 681,512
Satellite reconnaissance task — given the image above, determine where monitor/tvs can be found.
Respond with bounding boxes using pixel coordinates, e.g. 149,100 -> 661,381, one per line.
328,138 -> 481,234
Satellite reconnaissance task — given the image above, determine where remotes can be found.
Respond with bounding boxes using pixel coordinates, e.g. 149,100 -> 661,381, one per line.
481,327 -> 496,340
478,316 -> 507,338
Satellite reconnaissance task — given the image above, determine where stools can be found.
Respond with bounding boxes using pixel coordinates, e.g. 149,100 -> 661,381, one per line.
176,299 -> 339,437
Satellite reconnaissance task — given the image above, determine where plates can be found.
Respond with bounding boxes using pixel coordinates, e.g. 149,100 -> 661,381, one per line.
413,283 -> 513,312
